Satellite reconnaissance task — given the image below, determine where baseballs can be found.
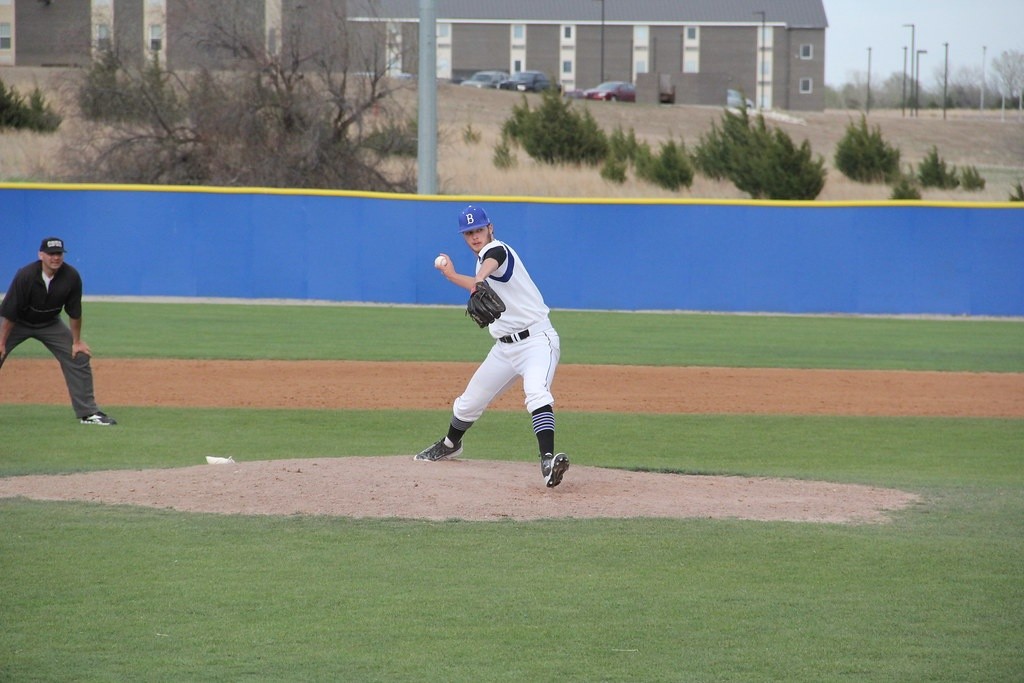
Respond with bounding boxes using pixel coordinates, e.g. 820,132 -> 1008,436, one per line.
434,256 -> 446,267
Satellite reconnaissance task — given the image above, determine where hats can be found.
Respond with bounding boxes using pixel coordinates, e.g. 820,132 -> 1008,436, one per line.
38,237 -> 67,255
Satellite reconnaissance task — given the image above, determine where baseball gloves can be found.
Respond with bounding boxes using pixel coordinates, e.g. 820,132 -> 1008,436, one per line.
464,279 -> 506,329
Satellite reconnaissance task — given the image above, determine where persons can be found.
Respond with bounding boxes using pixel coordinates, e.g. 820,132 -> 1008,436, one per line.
414,206 -> 570,488
0,238 -> 118,425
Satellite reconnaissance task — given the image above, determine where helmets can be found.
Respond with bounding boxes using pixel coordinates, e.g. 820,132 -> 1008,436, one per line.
457,204 -> 489,233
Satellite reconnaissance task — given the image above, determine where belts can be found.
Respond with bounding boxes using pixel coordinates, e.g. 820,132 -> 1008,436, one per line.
496,329 -> 530,344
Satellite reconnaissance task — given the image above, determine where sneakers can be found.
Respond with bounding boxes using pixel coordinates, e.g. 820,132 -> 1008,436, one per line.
541,453 -> 570,488
79,410 -> 117,426
414,436 -> 463,463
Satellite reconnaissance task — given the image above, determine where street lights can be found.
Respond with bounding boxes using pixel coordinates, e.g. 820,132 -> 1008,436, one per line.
942,42 -> 950,119
902,22 -> 915,116
915,48 -> 929,115
902,46 -> 908,117
865,47 -> 873,115
753,10 -> 768,111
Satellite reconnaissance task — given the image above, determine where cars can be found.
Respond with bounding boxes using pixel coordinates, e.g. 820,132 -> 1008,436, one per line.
583,81 -> 636,101
726,88 -> 753,110
497,71 -> 561,96
458,70 -> 510,89
